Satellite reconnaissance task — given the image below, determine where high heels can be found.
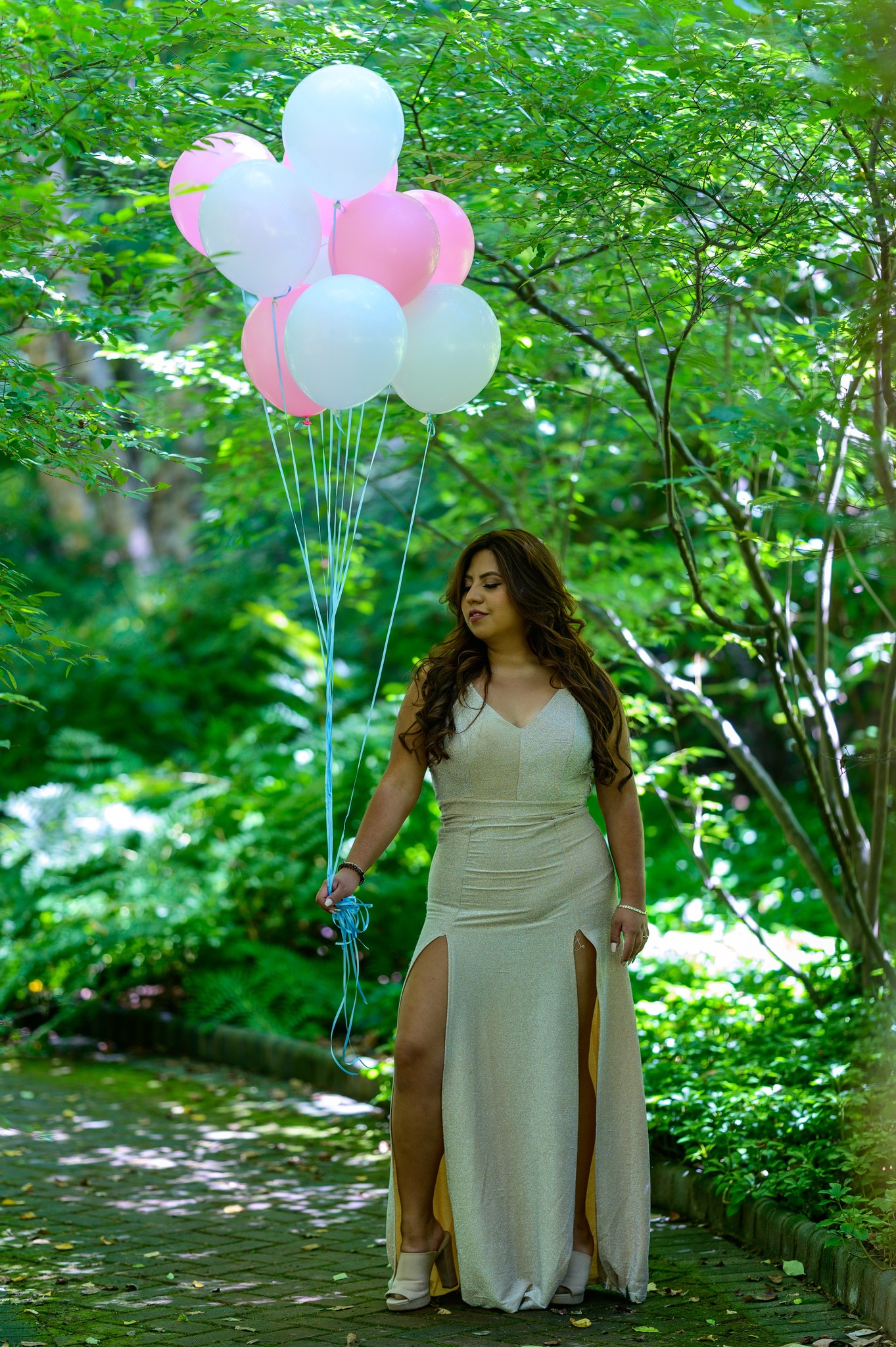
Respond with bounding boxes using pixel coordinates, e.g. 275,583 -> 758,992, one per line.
385,1231 -> 459,1311
550,1251 -> 592,1304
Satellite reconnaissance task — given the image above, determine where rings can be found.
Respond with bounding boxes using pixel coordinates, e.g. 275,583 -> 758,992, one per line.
642,934 -> 648,939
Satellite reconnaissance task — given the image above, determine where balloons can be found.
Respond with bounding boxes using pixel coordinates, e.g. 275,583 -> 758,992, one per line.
281,64 -> 405,208
392,282 -> 501,425
198,158 -> 323,304
402,188 -> 474,286
241,281 -> 326,426
284,273 -> 407,418
168,131 -> 277,257
282,150 -> 399,285
328,189 -> 441,308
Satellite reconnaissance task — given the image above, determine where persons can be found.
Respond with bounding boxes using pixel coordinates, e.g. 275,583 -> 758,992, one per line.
315,527 -> 651,1312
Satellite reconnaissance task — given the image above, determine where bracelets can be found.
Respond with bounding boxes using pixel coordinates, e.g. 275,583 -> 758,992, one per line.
617,905 -> 647,915
338,857 -> 365,886
